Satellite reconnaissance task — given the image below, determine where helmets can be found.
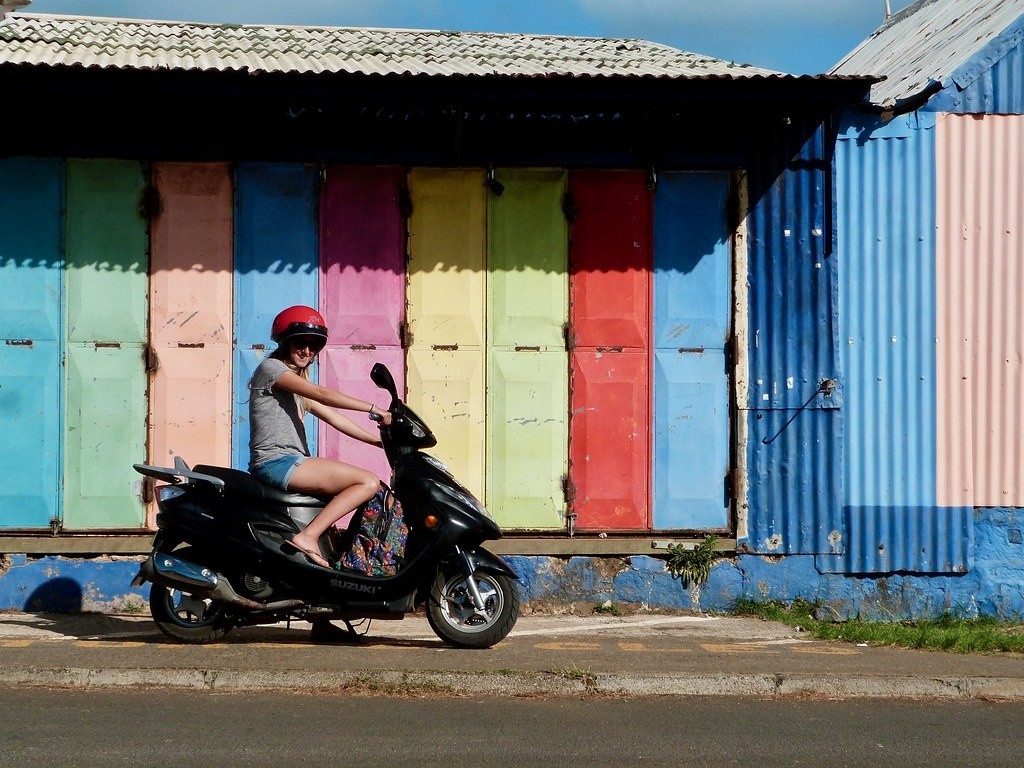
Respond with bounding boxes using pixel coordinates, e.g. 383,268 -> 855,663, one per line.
271,306 -> 328,356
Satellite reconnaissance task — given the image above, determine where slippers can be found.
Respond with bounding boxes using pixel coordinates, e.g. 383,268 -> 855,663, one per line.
285,540 -> 333,569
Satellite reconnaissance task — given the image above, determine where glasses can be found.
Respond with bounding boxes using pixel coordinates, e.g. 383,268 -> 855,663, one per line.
294,338 -> 321,351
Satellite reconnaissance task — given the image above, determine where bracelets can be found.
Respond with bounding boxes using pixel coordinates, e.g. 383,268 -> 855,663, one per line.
369,404 -> 374,413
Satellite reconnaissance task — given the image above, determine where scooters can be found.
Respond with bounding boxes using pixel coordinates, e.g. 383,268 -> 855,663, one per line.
132,363 -> 520,649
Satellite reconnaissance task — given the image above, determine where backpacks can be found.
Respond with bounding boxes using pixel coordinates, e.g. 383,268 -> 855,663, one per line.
328,480 -> 409,577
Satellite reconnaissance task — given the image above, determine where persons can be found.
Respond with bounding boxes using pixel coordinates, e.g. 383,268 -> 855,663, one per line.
249,304 -> 394,639
